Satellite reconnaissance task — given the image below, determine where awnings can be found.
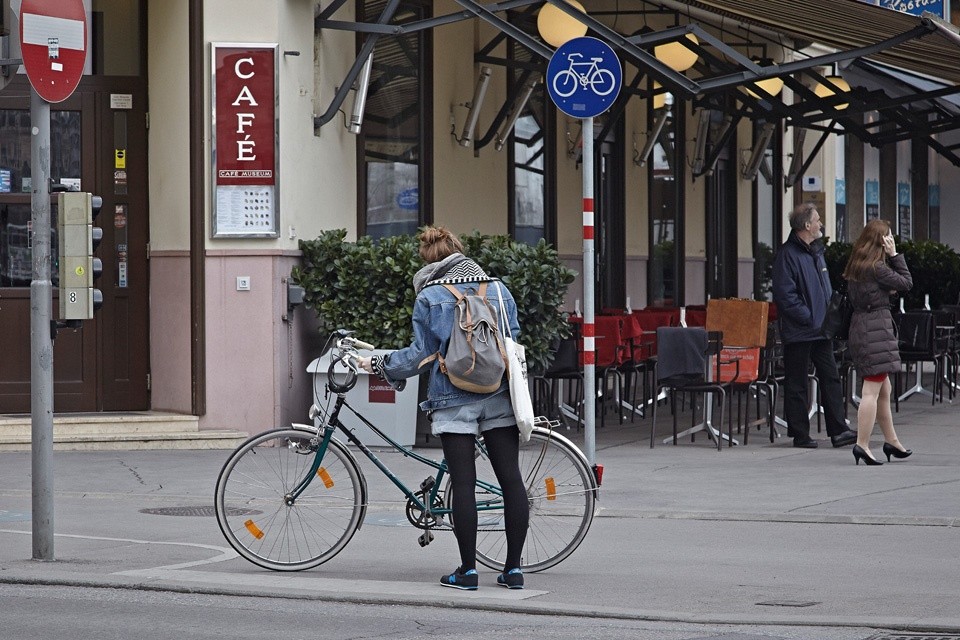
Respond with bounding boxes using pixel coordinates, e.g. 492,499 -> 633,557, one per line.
319,0 -> 960,186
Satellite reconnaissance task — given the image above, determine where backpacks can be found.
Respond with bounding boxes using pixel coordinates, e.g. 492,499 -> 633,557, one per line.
430,279 -> 507,396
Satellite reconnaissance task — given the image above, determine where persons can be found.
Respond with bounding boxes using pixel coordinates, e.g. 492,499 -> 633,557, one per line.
845,219 -> 912,465
771,203 -> 857,447
357,227 -> 530,591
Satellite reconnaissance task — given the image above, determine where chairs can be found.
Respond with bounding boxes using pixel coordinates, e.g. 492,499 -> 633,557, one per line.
541,303 -> 960,452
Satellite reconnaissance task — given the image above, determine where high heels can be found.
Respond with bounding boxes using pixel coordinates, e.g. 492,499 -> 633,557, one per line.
852,445 -> 887,465
882,442 -> 914,461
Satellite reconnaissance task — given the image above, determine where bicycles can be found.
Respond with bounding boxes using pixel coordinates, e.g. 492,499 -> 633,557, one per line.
214,328 -> 603,573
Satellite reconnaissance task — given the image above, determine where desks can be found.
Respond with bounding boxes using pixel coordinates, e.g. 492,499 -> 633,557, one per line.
557,333 -> 606,425
897,326 -> 954,403
662,345 -> 760,446
609,329 -> 656,418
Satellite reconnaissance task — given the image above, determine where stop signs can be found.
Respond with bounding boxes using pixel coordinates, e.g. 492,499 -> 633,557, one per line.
19,0 -> 87,104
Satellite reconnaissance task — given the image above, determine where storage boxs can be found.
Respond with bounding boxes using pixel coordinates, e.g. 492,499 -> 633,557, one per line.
707,296 -> 769,347
713,347 -> 761,383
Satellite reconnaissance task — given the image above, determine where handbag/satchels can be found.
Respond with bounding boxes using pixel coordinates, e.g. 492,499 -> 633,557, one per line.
493,282 -> 539,441
821,289 -> 862,344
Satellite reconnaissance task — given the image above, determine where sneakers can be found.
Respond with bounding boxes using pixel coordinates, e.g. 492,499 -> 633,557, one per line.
439,568 -> 481,591
495,571 -> 525,588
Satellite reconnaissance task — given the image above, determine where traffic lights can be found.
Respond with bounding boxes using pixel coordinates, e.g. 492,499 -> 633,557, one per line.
59,192 -> 103,327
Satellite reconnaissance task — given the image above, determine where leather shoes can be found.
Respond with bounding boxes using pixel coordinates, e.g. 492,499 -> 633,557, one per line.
793,438 -> 821,447
831,430 -> 860,449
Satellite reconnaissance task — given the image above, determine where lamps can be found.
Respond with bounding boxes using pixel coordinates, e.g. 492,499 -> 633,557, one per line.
537,0 -> 587,49
702,42 -> 785,99
814,61 -> 852,111
586,9 -> 699,73
